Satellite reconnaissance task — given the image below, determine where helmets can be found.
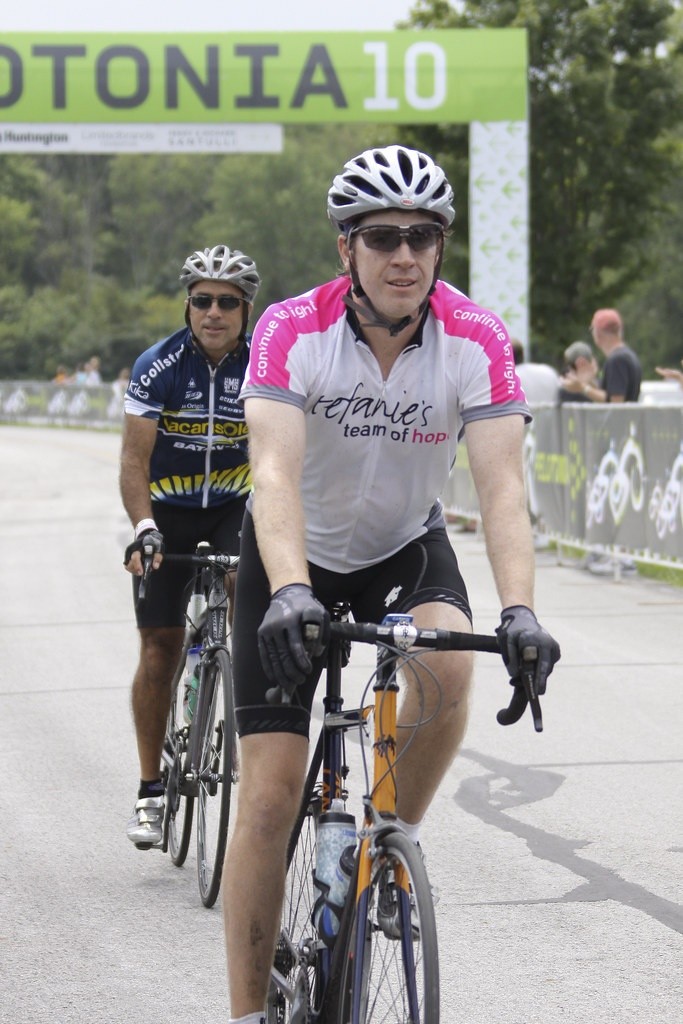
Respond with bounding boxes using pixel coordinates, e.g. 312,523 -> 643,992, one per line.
322,145 -> 456,229
177,245 -> 263,305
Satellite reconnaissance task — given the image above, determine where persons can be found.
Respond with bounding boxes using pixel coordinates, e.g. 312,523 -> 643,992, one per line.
119,247 -> 261,843
228,146 -> 562,1023
512,311 -> 683,576
1,355 -> 133,418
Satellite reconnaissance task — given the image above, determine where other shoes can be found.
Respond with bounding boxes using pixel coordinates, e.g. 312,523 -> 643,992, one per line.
371,855 -> 422,944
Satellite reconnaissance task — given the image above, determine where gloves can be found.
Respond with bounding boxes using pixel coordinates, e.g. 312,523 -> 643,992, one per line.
258,582 -> 327,690
498,606 -> 560,696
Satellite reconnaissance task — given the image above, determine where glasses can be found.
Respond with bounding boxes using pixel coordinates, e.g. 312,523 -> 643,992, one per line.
351,222 -> 444,255
184,293 -> 247,311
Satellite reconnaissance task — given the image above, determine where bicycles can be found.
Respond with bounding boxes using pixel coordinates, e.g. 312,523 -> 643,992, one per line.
135,540 -> 241,909
266,601 -> 544,1023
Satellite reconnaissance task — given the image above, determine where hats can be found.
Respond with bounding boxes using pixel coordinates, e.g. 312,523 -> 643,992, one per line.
588,311 -> 619,333
564,342 -> 590,365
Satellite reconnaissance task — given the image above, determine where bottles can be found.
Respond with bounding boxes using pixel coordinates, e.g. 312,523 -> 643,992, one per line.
184,648 -> 202,724
315,798 -> 357,936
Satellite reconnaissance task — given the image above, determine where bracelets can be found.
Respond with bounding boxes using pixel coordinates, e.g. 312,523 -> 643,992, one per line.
133,518 -> 158,537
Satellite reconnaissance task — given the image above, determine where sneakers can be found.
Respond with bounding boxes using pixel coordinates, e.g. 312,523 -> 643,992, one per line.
126,795 -> 165,842
